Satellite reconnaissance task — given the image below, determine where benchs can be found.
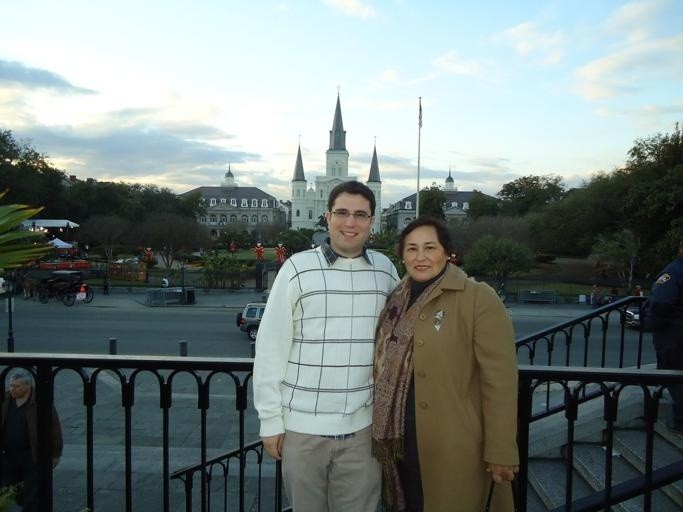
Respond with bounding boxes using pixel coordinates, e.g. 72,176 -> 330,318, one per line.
519,288 -> 557,305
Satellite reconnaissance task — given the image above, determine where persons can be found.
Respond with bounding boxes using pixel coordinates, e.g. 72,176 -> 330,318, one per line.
250,180 -> 401,511
0,370 -> 62,510
633,284 -> 643,296
648,248 -> 682,436
611,284 -> 619,294
372,214 -> 522,510
593,283 -> 601,305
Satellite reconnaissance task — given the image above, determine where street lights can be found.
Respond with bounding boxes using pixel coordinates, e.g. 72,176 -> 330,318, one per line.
31,220 -> 36,231
1,271 -> 16,352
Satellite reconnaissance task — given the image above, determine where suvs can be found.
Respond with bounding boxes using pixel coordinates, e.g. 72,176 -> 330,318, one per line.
235,302 -> 267,342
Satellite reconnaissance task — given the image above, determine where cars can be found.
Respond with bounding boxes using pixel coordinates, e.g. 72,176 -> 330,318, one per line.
620,302 -> 653,332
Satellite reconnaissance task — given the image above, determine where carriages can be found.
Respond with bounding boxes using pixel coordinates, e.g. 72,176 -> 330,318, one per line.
10,268 -> 93,307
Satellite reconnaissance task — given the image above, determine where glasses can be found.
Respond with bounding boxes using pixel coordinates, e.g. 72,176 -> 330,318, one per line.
331,208 -> 370,223
